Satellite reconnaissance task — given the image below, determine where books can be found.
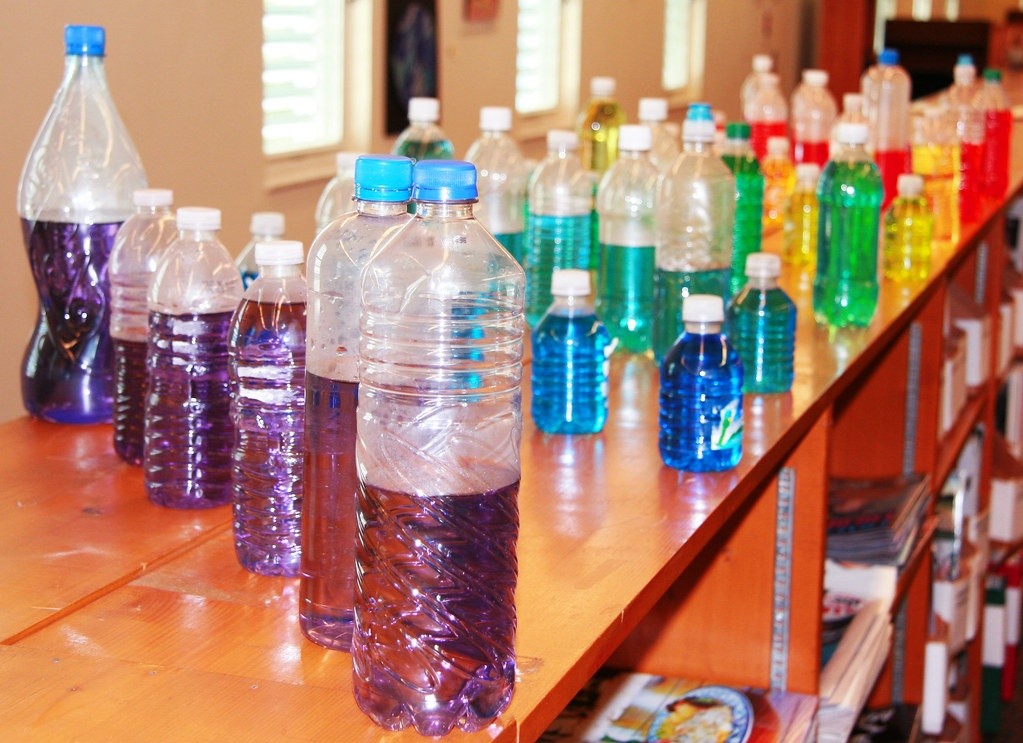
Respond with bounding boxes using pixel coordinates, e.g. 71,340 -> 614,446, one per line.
539,288 -> 1023,743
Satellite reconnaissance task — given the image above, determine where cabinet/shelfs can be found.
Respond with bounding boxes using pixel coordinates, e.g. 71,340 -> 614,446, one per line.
0,112 -> 1023,743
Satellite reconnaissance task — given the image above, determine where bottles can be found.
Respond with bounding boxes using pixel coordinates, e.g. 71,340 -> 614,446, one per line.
238,213 -> 287,291
141,206 -> 239,509
294,155 -> 418,650
348,161 -> 523,737
226,240 -> 314,578
107,190 -> 182,465
379,47 -> 1012,473
15,25 -> 162,424
